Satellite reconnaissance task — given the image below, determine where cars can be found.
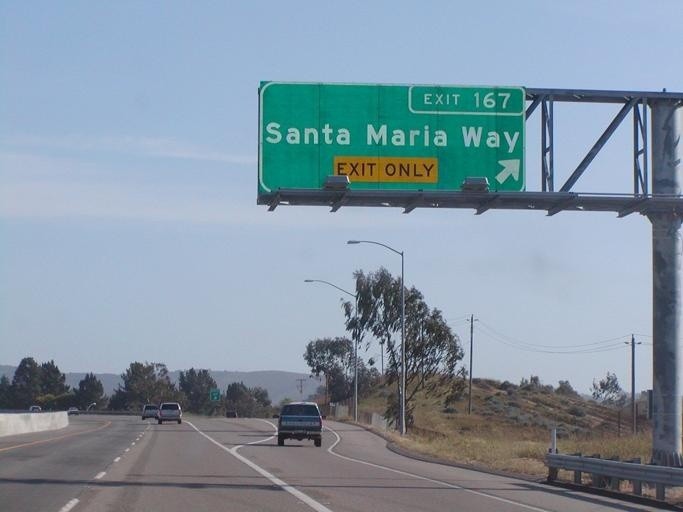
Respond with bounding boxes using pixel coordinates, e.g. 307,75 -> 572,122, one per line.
67,407 -> 79,415
29,406 -> 41,412
142,402 -> 180,424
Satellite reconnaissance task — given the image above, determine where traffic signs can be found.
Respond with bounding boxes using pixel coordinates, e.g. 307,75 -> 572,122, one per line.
259,79 -> 527,195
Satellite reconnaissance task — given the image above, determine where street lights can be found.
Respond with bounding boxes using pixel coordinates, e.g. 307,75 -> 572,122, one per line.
304,279 -> 358,421
347,239 -> 406,435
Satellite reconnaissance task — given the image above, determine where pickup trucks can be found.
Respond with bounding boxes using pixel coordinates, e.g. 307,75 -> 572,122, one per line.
277,401 -> 322,445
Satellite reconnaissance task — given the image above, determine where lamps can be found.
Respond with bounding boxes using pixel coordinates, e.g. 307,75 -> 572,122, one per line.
461,176 -> 489,190
323,174 -> 350,190
